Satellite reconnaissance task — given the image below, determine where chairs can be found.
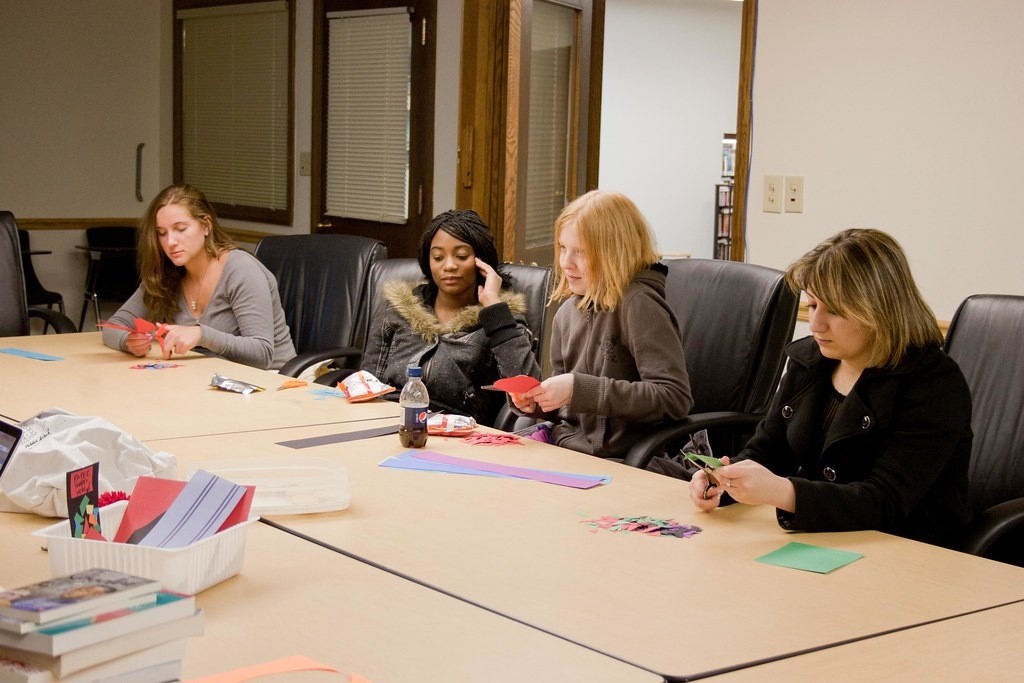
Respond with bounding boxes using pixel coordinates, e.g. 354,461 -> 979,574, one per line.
310,246 -> 551,428
489,252 -> 805,466
916,284 -> 1024,567
18,227 -> 67,335
245,229 -> 389,379
0,209 -> 78,337
74,222 -> 148,336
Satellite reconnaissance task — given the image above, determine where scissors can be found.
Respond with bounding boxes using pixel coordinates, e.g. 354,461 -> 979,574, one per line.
149,323 -> 173,355
680,433 -> 721,501
480,374 -> 528,404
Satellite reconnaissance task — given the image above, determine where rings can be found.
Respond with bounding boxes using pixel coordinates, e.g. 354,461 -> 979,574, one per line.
725,479 -> 731,486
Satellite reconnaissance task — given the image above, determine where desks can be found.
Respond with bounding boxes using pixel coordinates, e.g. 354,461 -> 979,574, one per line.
0,325 -> 1024,683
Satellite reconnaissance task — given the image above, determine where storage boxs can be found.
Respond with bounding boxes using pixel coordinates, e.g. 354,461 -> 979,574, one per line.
28,495 -> 262,594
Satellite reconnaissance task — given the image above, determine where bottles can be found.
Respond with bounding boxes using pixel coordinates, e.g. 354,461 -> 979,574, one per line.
398,366 -> 429,448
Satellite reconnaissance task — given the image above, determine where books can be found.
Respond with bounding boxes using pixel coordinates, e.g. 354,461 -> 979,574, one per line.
0,566 -> 205,683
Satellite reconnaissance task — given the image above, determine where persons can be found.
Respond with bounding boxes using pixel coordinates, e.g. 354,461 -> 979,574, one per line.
690,227 -> 974,551
102,184 -> 297,374
360,209 -> 543,428
506,184 -> 693,475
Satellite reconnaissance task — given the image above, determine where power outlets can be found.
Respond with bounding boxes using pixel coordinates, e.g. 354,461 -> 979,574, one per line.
784,175 -> 804,215
761,176 -> 784,213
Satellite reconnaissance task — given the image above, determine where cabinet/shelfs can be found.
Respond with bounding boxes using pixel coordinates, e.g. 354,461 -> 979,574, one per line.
712,181 -> 734,262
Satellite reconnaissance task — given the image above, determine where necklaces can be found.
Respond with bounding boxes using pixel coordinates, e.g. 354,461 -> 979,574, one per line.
184,256 -> 213,308
